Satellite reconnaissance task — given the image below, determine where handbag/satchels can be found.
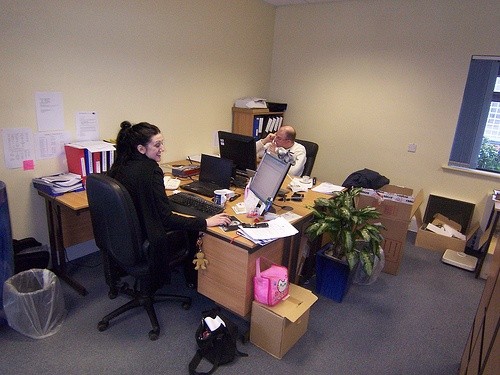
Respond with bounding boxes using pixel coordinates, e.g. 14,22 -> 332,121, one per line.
188,309 -> 248,375
254,255 -> 290,306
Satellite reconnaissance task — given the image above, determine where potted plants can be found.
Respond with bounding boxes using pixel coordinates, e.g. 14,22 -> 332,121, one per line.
304,188 -> 387,303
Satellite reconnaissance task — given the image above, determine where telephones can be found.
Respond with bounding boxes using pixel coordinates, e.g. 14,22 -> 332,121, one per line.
163,176 -> 181,190
273,133 -> 276,144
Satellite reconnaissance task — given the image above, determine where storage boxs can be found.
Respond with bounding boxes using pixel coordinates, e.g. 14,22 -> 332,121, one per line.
248,281 -> 319,359
352,183 -> 481,275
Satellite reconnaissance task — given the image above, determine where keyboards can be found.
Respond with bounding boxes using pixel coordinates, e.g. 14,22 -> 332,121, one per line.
167,192 -> 224,219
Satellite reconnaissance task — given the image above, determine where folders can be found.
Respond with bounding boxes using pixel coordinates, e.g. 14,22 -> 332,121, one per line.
253,116 -> 283,141
65,145 -> 115,185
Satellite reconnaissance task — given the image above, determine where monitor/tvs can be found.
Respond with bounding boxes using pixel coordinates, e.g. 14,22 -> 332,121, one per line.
249,152 -> 292,216
218,131 -> 257,172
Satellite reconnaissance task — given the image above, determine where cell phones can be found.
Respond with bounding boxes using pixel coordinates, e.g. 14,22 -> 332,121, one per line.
291,193 -> 304,200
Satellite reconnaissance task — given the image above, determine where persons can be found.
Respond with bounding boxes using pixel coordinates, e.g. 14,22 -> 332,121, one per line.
106,121 -> 231,289
256,125 -> 307,177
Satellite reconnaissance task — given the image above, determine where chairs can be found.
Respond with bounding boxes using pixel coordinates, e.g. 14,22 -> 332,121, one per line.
86,172 -> 191,341
294,138 -> 319,177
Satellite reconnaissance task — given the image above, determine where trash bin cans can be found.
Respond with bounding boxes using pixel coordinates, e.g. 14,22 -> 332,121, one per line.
352,240 -> 380,285
6,269 -> 62,338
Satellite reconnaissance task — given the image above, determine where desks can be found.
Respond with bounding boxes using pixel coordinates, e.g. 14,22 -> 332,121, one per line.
38,159 -> 347,323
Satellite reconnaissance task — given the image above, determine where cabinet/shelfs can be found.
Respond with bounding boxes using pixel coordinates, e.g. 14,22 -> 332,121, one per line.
232,106 -> 285,142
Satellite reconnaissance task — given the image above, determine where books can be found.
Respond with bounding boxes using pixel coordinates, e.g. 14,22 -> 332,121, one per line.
32,140 -> 116,196
254,115 -> 283,139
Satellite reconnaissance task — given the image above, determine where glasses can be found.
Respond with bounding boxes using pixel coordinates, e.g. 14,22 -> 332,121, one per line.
275,133 -> 289,141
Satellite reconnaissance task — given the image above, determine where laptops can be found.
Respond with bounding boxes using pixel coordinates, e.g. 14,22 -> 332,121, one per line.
179,154 -> 233,198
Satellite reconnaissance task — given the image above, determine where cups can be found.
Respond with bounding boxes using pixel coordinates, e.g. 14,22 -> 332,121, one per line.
213,190 -> 227,207
303,176 -> 310,183
